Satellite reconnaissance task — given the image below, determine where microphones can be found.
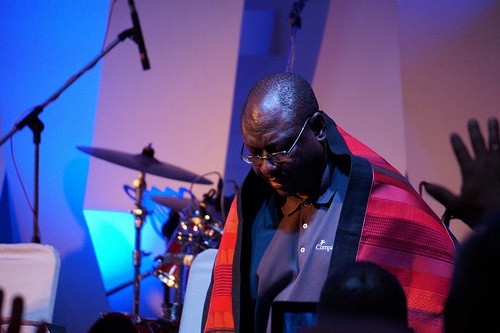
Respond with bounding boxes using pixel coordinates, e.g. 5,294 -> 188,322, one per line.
288,0 -> 307,19
217,178 -> 226,225
127,0 -> 150,71
198,195 -> 211,215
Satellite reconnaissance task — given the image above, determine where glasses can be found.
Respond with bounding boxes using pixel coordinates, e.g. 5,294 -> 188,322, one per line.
240,111 -> 323,166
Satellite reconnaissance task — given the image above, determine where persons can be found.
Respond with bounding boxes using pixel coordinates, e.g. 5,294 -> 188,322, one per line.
318,114 -> 500,333
202,72 -> 455,333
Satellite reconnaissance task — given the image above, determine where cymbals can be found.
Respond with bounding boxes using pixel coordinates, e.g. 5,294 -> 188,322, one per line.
76,145 -> 214,185
150,196 -> 226,223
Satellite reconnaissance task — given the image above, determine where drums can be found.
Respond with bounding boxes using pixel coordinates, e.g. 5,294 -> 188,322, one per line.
85,311 -> 180,333
153,211 -> 225,291
